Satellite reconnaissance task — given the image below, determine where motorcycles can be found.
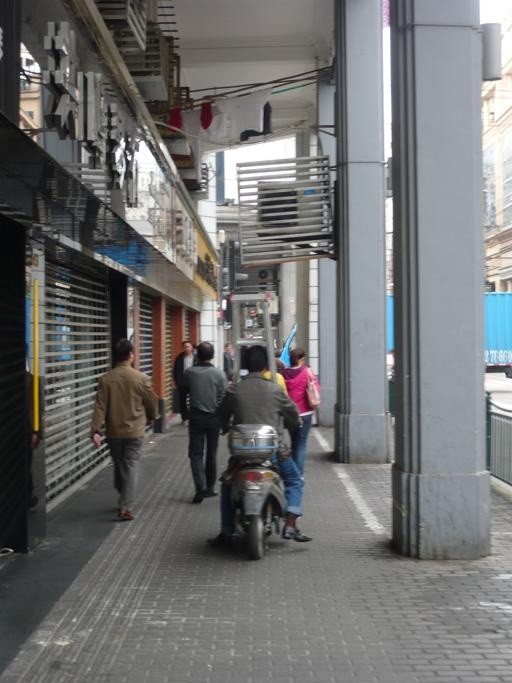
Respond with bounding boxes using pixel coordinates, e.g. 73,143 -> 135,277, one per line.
220,414 -> 304,560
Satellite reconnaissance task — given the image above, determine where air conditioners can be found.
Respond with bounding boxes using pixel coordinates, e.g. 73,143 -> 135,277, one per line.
256,269 -> 274,292
258,181 -> 322,235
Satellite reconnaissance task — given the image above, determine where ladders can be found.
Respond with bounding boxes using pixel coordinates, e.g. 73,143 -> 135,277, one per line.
228,291 -> 276,384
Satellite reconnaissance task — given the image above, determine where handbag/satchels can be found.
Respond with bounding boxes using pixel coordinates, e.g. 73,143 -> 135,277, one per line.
306,367 -> 321,408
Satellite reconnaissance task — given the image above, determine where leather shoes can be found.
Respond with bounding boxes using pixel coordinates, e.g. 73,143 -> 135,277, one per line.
117,508 -> 135,519
283,526 -> 313,542
193,491 -> 218,503
207,533 -> 232,547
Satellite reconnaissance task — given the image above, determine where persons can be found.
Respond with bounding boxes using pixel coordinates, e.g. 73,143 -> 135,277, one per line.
91,338 -> 161,520
179,341 -> 229,503
173,341 -> 289,426
282,347 -> 321,481
206,345 -> 313,548
